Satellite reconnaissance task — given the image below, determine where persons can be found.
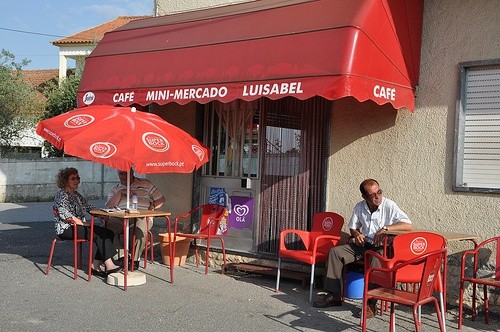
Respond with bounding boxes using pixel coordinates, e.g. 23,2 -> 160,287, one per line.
312,179 -> 412,318
105,168 -> 165,271
52,168 -> 122,278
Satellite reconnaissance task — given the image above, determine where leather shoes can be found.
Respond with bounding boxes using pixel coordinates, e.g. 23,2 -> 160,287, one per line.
313,294 -> 342,307
357,303 -> 379,318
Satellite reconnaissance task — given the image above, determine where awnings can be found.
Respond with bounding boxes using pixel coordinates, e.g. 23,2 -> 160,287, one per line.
76,0 -> 424,113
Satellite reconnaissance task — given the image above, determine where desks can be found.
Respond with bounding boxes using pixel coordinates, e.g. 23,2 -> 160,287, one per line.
90,208 -> 172,291
380,231 -> 479,321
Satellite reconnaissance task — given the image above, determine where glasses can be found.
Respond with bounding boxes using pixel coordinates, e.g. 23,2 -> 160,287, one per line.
366,189 -> 383,198
68,177 -> 80,181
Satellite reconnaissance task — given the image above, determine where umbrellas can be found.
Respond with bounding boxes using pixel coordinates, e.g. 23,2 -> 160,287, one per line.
36,105 -> 210,270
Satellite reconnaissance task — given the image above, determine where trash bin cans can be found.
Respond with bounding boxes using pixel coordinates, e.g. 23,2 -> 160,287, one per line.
346,263 -> 364,299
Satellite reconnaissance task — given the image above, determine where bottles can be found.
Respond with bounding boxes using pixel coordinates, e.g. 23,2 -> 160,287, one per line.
131,193 -> 139,209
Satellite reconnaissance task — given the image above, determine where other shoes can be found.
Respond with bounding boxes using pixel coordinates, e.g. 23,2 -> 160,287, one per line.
92,268 -> 107,277
105,265 -> 124,274
128,261 -> 139,270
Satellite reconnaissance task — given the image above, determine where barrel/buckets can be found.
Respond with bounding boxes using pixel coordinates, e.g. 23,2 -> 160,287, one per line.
345,272 -> 367,299
157,232 -> 192,266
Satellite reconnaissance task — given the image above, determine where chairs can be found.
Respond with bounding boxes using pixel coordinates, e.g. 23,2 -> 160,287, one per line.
276,212 -> 500,332
45,203 -> 227,284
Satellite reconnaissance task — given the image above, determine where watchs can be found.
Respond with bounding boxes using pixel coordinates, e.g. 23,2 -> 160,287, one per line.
382,227 -> 388,231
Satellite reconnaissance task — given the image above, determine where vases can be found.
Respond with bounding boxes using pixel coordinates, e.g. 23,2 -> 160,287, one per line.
159,232 -> 192,265
